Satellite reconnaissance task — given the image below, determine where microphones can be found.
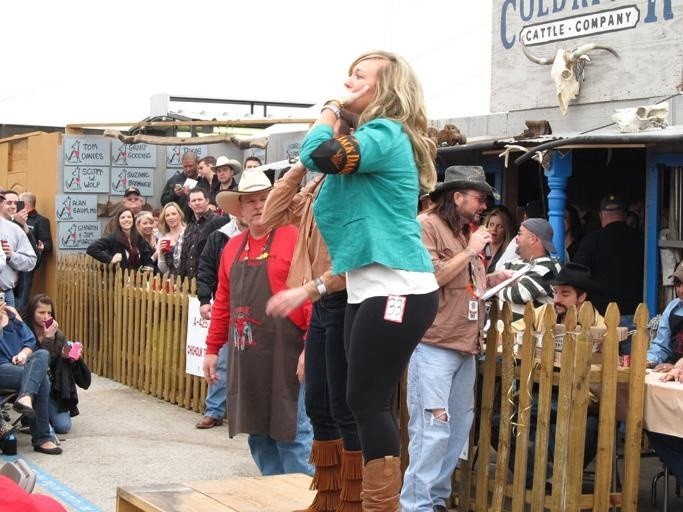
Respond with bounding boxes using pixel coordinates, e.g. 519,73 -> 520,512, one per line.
478,216 -> 492,260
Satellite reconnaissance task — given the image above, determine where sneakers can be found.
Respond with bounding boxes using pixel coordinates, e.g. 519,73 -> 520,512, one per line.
32,445 -> 63,454
11,400 -> 36,418
194,415 -> 223,429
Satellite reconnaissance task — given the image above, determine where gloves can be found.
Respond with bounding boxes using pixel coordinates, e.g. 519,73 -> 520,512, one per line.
110,253 -> 122,264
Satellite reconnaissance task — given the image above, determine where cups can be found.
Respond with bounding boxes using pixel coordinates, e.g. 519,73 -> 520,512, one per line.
162,239 -> 171,253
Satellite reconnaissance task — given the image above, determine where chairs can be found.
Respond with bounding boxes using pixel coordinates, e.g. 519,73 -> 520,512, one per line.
1,389 -> 61,446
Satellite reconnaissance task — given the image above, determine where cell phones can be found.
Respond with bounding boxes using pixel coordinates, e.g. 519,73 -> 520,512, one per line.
163,240 -> 170,253
43,316 -> 54,328
175,184 -> 181,190
17,201 -> 24,213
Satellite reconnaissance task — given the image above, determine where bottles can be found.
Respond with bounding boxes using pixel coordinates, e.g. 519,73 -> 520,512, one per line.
61,341 -> 83,361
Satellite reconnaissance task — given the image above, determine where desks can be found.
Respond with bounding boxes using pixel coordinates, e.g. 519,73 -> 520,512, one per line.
476,344 -> 683,511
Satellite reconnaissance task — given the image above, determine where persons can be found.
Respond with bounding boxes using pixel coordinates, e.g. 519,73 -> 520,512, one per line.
0,188 -> 92,455
83,151 -> 264,430
261,116 -> 361,512
204,169 -> 315,477
400,164 -> 682,512
299,51 -> 439,511
0,474 -> 65,511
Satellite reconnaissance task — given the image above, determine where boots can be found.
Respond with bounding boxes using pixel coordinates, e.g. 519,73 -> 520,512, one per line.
291,438 -> 404,510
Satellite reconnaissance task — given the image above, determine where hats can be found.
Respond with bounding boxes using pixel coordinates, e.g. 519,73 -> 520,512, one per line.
419,165 -> 501,201
215,168 -> 275,219
599,192 -> 627,212
521,218 -> 558,254
667,260 -> 683,284
209,156 -> 243,176
123,187 -> 140,197
541,262 -> 597,289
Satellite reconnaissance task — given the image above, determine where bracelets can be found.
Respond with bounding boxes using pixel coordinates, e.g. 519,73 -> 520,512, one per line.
321,98 -> 344,119
